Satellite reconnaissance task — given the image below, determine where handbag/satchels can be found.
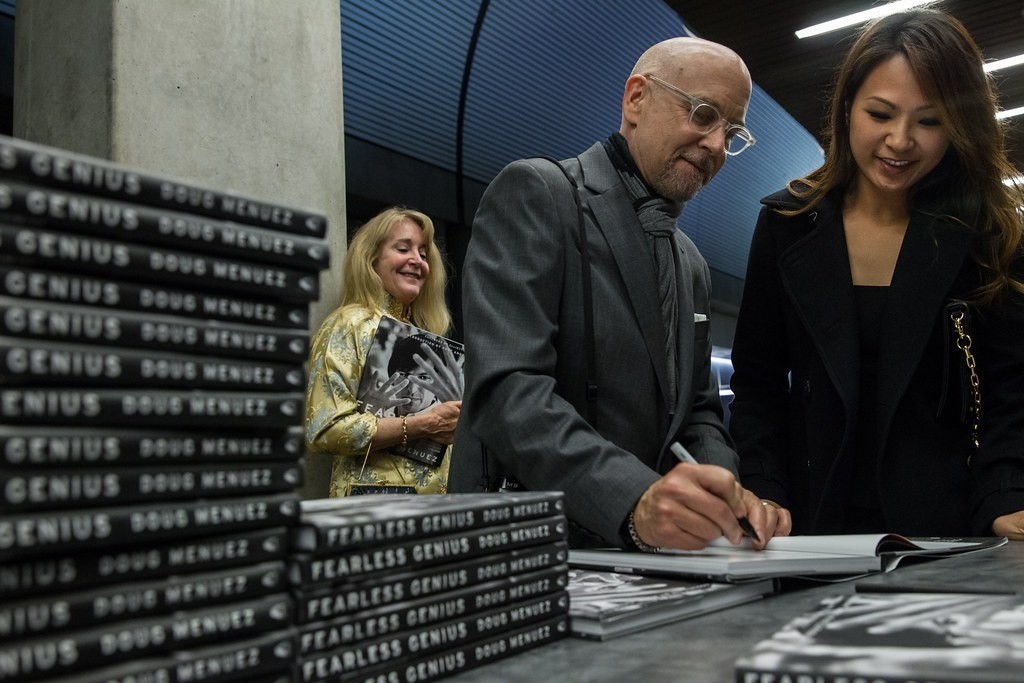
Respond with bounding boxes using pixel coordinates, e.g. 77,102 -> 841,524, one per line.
342,437 -> 419,496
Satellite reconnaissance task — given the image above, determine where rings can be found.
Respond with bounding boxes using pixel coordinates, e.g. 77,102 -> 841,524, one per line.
760,501 -> 770,508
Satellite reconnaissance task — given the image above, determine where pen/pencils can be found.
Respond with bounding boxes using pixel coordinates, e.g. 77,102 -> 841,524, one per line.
670,441 -> 761,543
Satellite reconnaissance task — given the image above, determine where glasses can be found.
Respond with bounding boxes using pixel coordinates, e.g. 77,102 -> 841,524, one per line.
644,74 -> 757,157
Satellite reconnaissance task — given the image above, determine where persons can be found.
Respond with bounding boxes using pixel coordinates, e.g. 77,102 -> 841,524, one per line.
740,591 -> 1024,667
357,334 -> 464,418
445,36 -> 794,556
303,205 -> 469,500
729,8 -> 1024,540
567,568 -> 727,615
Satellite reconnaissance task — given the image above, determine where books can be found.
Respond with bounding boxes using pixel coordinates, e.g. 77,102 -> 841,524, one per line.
1,132 -> 330,683
292,486 -> 568,683
353,313 -> 467,469
561,525 -> 1002,640
733,578 -> 1024,683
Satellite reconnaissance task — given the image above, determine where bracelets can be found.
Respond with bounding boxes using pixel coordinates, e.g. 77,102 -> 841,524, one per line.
401,416 -> 408,448
626,508 -> 662,553
